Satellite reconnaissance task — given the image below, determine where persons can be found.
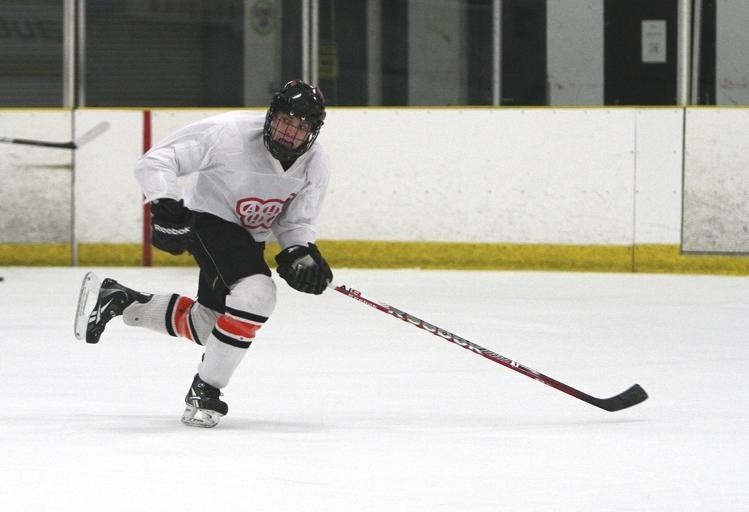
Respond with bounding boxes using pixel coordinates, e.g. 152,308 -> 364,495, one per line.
85,79 -> 333,416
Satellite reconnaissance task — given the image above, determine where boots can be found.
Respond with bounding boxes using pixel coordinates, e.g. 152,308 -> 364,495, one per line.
86,278 -> 154,344
185,354 -> 228,416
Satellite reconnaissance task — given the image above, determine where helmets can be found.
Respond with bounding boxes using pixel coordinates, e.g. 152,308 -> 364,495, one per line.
265,80 -> 326,163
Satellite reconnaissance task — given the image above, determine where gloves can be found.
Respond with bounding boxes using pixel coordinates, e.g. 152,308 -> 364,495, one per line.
274,242 -> 333,294
149,199 -> 191,255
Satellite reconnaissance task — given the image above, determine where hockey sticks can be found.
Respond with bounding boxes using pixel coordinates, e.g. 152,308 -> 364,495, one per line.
326,279 -> 649,411
